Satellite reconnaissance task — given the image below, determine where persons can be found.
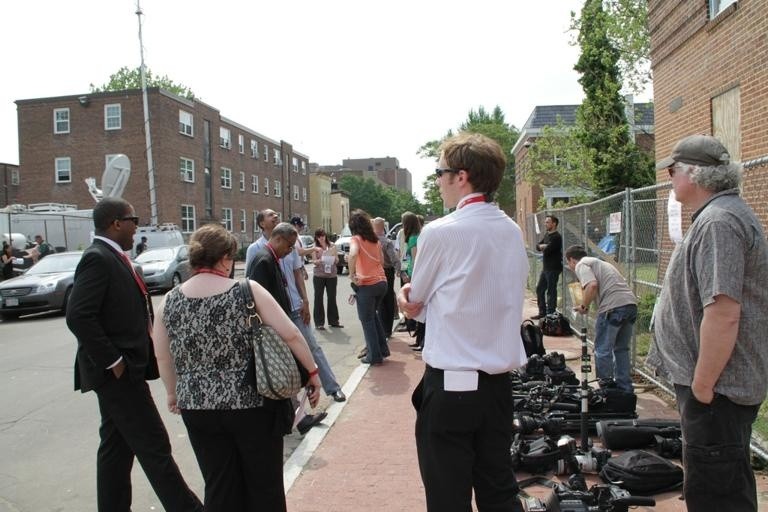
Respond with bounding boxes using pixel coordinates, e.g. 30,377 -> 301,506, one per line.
135,235 -> 148,256
63,195 -> 206,512
347,207 -> 427,364
0,231 -> 51,281
150,223 -> 322,512
395,131 -> 530,512
566,244 -> 638,394
245,209 -> 347,434
642,132 -> 768,512
531,215 -> 563,319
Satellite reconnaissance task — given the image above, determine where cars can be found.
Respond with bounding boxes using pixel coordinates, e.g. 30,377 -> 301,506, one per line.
0,251 -> 143,318
298,233 -> 314,264
133,244 -> 197,291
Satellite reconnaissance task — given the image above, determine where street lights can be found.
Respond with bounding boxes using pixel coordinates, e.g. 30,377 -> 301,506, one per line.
134,1 -> 160,229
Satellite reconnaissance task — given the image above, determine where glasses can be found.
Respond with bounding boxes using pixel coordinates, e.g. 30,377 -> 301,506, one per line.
435,168 -> 467,178
114,217 -> 138,225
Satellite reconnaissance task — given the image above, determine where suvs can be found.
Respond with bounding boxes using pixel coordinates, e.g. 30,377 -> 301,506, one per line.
334,218 -> 403,275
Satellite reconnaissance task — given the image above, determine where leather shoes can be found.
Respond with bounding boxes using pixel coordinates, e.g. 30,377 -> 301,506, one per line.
397,321 -> 422,351
330,390 -> 345,402
296,411 -> 327,435
357,345 -> 391,365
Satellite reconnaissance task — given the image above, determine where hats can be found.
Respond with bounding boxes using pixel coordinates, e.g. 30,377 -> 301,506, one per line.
291,216 -> 306,226
654,134 -> 730,172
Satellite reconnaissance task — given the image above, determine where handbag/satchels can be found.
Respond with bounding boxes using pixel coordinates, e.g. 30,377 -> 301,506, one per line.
598,450 -> 683,495
538,312 -> 574,337
252,325 -> 301,401
588,389 -> 637,413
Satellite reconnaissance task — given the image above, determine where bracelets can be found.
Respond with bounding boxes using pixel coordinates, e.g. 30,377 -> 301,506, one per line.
580,303 -> 589,314
306,365 -> 322,378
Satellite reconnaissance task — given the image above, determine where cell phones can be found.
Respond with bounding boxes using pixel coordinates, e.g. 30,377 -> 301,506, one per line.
307,385 -> 315,408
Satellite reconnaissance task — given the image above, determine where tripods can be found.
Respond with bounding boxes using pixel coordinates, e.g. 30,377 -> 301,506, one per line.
523,412 -> 639,434
